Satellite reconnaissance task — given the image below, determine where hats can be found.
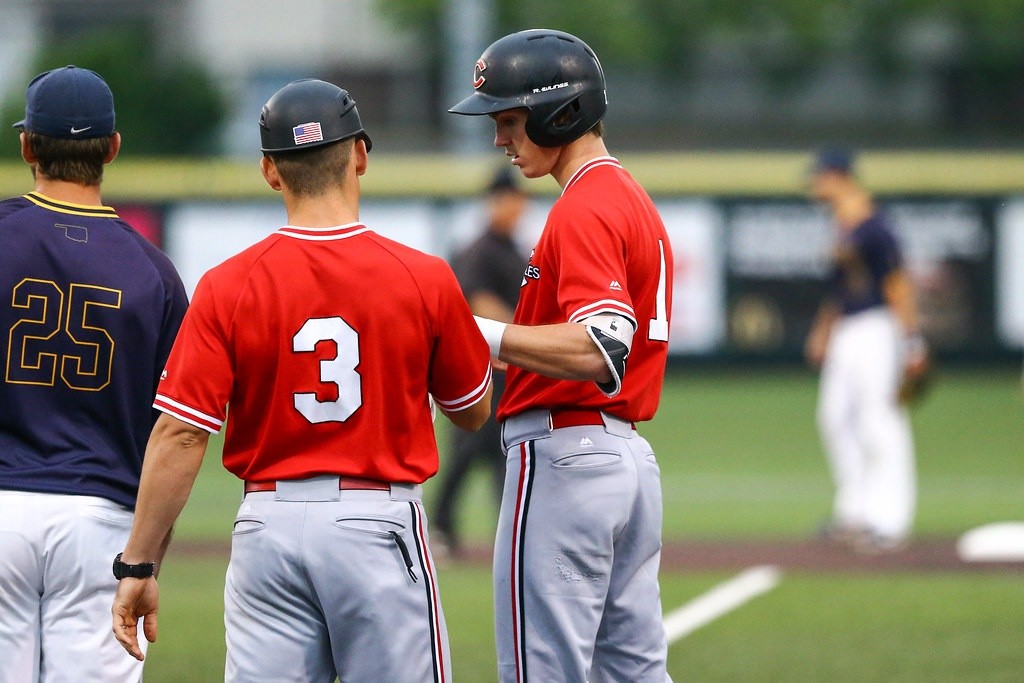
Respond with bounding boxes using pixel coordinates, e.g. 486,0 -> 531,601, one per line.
13,65 -> 116,136
810,141 -> 851,175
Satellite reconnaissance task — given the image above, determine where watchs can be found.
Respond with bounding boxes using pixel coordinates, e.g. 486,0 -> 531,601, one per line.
113,552 -> 155,580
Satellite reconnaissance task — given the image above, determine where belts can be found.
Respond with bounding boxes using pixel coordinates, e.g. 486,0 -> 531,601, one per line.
548,406 -> 636,431
244,474 -> 391,493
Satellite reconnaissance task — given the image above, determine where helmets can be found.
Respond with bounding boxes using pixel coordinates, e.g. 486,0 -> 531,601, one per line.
449,27 -> 607,146
258,78 -> 373,153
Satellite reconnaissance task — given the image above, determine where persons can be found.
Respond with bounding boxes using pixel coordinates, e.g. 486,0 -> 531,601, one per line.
424,162 -> 529,560
0,64 -> 190,683
800,141 -> 929,557
449,28 -> 681,683
920,254 -> 993,368
110,80 -> 494,683
